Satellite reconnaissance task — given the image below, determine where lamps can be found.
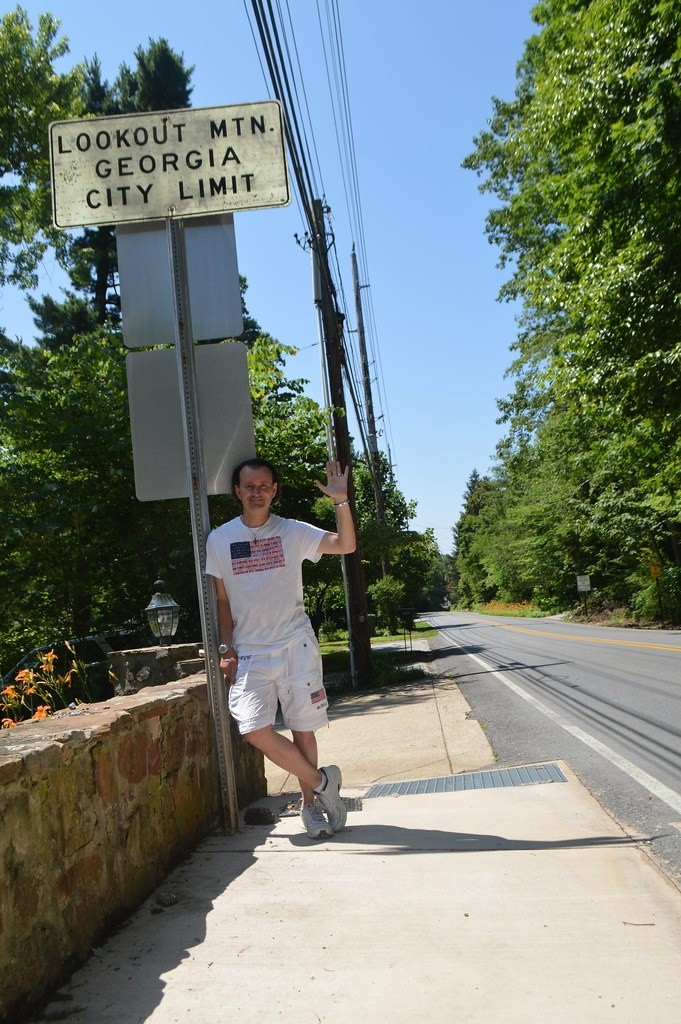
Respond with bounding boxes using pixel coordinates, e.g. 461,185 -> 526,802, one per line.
145,572 -> 181,647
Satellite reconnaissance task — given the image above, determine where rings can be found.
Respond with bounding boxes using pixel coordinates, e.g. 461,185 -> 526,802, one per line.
337,473 -> 342,477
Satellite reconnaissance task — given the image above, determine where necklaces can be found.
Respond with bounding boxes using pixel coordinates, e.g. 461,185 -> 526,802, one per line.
241,513 -> 270,545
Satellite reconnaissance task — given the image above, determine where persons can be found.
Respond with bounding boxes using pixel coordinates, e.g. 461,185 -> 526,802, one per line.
205,457 -> 357,840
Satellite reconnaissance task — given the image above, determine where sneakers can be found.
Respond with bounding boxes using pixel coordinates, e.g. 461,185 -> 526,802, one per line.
301,805 -> 334,839
313,765 -> 347,833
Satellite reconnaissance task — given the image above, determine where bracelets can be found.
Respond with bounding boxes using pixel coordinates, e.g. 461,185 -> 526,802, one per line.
333,500 -> 350,508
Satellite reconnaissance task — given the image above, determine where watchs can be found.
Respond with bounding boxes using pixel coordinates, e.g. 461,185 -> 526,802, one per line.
218,643 -> 233,654
